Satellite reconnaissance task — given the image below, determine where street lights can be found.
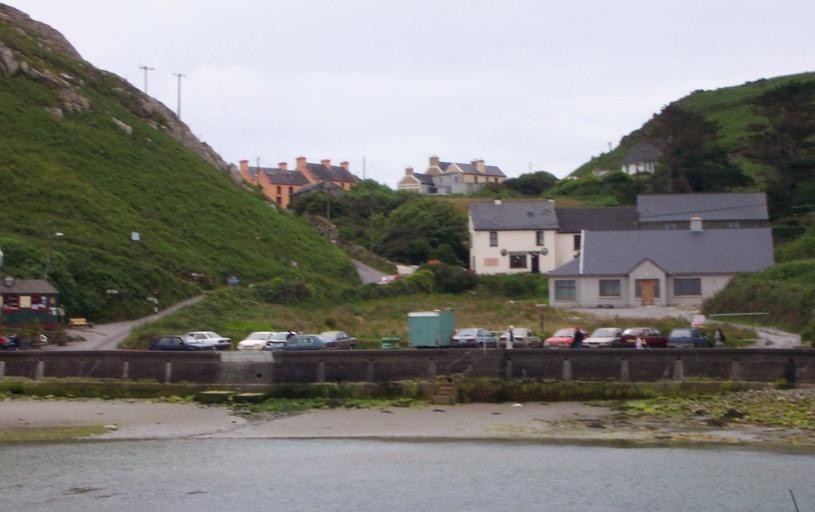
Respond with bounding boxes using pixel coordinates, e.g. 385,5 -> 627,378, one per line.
138,65 -> 154,95
44,232 -> 65,281
172,71 -> 187,120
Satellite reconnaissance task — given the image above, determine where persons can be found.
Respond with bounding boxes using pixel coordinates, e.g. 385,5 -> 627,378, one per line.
286,329 -> 295,339
713,328 -> 727,347
58,303 -> 66,323
505,324 -> 515,350
636,334 -> 647,349
574,327 -> 583,349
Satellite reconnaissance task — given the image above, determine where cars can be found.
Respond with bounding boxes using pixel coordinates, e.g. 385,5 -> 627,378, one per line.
542,327 -> 588,348
581,327 -> 624,349
620,327 -> 669,348
224,273 -> 242,287
500,327 -> 542,349
0,332 -> 48,351
665,327 -> 711,349
451,328 -> 497,349
151,329 -> 359,352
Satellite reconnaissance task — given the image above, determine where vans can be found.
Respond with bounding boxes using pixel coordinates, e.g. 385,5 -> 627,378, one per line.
378,273 -> 401,286
